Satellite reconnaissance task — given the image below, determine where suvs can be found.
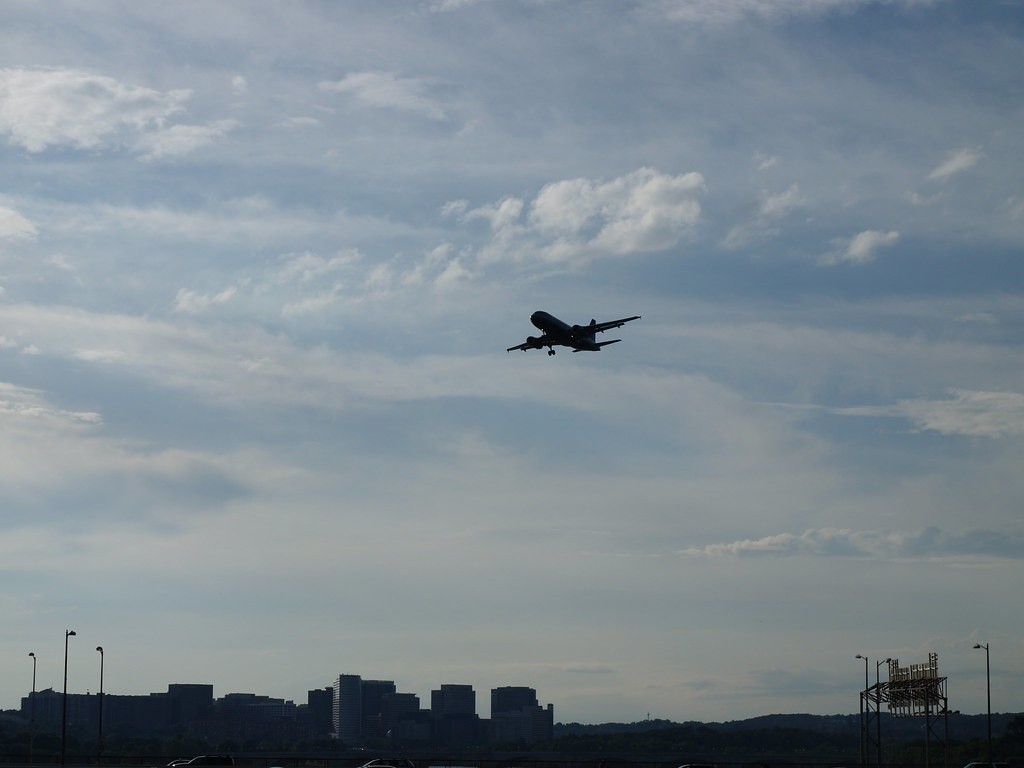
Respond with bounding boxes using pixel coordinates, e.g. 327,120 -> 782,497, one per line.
355,757 -> 416,768
172,754 -> 236,768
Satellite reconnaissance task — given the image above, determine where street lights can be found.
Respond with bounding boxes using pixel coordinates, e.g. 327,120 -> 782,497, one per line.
61,629 -> 77,768
854,655 -> 870,768
972,642 -> 992,763
95,646 -> 104,765
29,652 -> 36,765
876,658 -> 893,767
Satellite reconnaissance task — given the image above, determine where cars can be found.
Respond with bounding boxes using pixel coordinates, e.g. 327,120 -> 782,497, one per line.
678,763 -> 720,768
963,762 -> 1010,768
166,759 -> 191,765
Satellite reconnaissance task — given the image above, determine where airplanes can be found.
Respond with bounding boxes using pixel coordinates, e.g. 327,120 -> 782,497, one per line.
507,310 -> 641,357
